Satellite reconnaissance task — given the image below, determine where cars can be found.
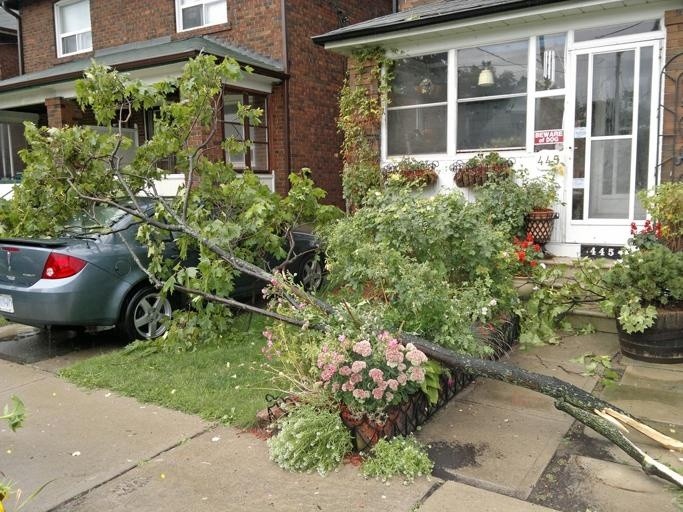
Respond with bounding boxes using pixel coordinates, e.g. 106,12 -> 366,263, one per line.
0,198 -> 327,341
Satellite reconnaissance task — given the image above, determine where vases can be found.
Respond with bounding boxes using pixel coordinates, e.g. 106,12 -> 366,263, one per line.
513,275 -> 537,300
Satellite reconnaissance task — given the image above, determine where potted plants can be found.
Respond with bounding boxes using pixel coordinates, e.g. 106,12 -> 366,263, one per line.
600,180 -> 683,364
382,150 -> 560,258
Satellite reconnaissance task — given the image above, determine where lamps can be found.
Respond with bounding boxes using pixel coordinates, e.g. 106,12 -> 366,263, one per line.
478,47 -> 494,87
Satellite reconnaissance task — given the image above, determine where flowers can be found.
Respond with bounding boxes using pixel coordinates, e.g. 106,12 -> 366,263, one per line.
510,232 -> 543,275
